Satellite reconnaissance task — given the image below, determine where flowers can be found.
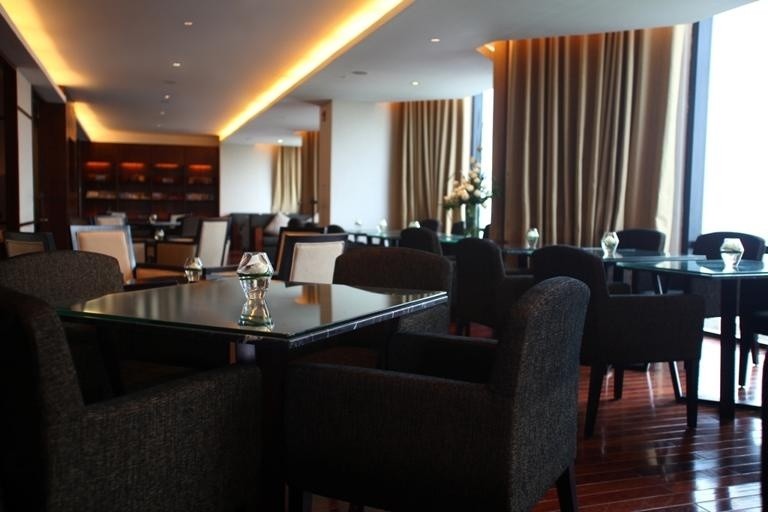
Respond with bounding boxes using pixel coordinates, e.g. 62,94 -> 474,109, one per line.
459,142 -> 498,234
437,177 -> 465,208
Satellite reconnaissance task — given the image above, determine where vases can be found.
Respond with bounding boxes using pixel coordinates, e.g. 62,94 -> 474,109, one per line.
463,202 -> 479,235
450,205 -> 462,234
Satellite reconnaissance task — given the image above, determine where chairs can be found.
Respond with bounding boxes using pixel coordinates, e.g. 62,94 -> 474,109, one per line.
0,250 -> 124,328
695,234 -> 764,264
328,248 -> 454,334
96,214 -> 153,265
204,230 -> 347,289
1,229 -> 55,252
69,223 -> 185,289
615,229 -> 663,253
398,227 -> 443,254
171,213 -> 204,242
419,217 -> 439,230
230,210 -> 245,263
280,276 -> 588,512
152,219 -> 229,265
247,211 -> 309,262
533,247 -> 707,433
447,238 -> 533,332
0,297 -> 270,511
